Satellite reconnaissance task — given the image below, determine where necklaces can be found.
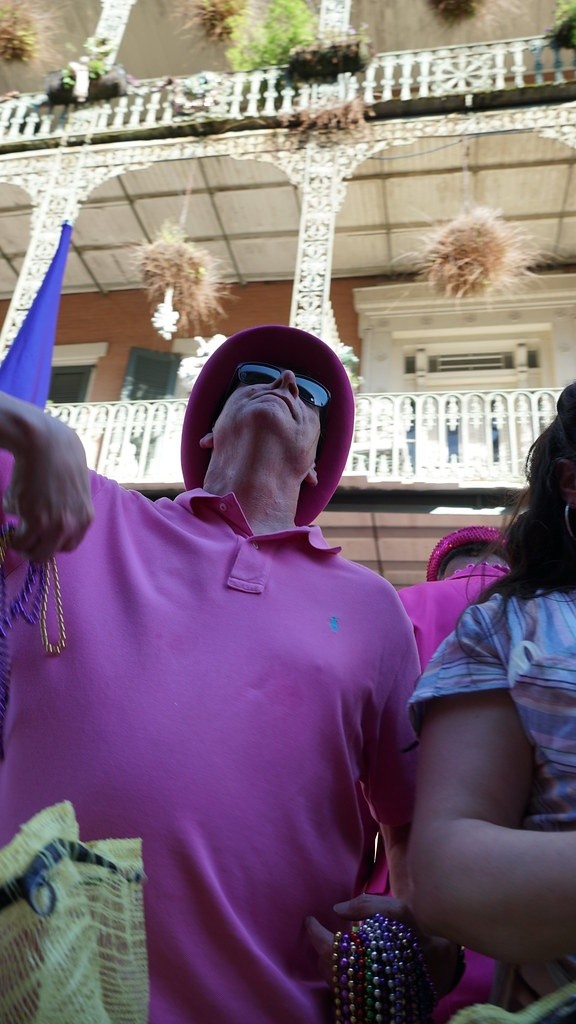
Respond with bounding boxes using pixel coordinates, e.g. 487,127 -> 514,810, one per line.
333,913 -> 438,1024
0,522 -> 66,726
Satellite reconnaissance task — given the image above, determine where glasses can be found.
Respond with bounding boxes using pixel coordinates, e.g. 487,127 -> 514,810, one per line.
440,541 -> 510,574
234,359 -> 332,408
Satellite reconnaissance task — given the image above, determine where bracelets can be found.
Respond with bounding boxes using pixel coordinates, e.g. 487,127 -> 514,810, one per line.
444,945 -> 465,993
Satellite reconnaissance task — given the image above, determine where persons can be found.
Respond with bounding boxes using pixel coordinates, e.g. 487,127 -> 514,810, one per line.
1,325 -> 466,1024
407,380 -> 576,1024
369,526 -> 513,1024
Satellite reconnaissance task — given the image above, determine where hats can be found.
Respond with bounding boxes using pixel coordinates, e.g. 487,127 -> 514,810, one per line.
181,325 -> 355,528
426,524 -> 513,582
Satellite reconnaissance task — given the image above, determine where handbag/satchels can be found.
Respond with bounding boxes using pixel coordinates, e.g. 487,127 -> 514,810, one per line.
330,914 -> 467,1024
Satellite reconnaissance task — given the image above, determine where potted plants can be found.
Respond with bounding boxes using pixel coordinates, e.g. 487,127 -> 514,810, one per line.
46,35 -> 127,106
